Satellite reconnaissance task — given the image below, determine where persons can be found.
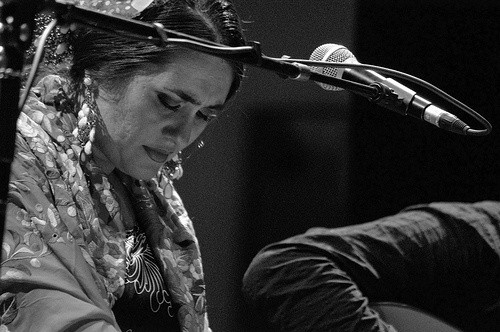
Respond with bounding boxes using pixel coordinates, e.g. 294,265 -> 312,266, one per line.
242,200 -> 500,332
0,0 -> 247,332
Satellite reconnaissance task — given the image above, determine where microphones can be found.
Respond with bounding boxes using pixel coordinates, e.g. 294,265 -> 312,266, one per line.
309,43 -> 470,136
